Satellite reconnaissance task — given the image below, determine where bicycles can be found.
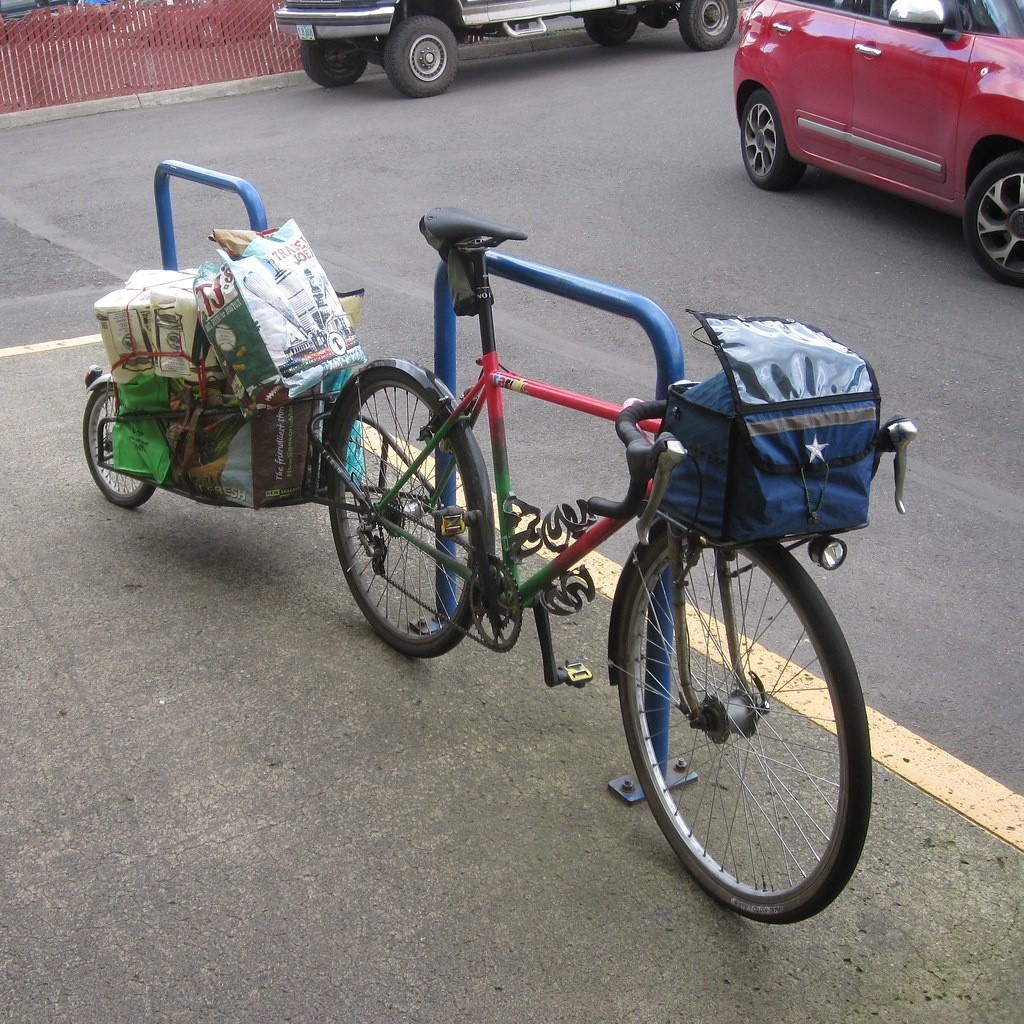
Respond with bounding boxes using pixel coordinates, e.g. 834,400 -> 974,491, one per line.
322,209 -> 874,927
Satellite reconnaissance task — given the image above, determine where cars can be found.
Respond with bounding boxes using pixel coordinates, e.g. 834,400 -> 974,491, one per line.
274,0 -> 738,97
734,0 -> 1024,288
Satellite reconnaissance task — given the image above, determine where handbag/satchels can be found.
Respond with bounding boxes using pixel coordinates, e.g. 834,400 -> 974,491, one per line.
193,219 -> 368,417
